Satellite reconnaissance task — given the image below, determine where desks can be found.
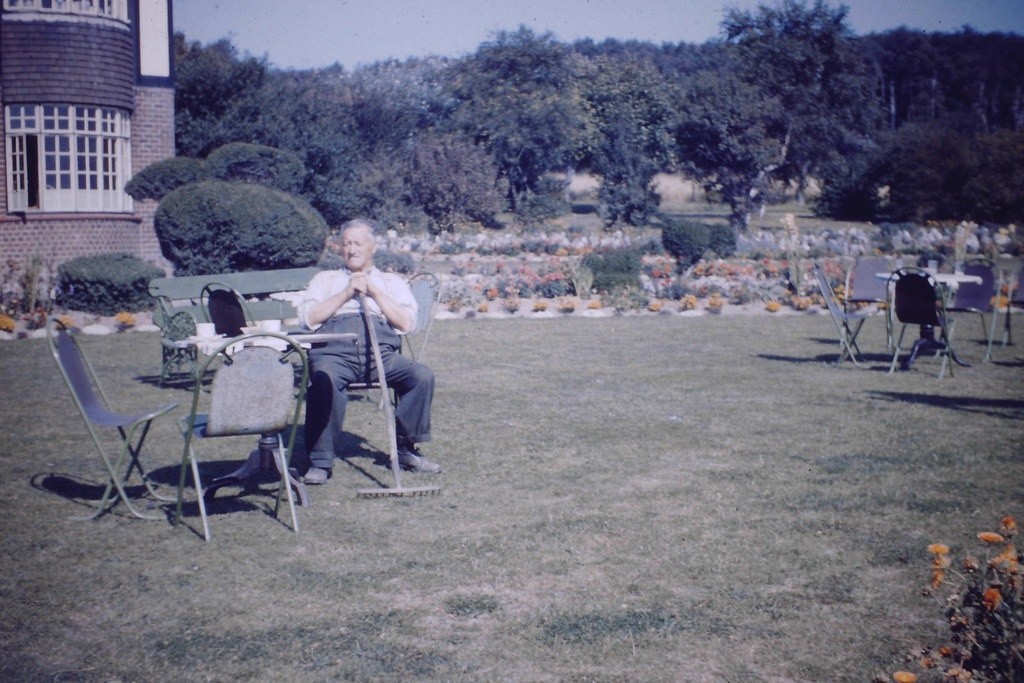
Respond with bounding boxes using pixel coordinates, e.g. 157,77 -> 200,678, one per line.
159,332 -> 358,511
875,272 -> 982,369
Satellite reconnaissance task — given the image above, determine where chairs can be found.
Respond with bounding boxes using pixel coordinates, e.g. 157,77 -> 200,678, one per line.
813,254 -> 1024,381
200,283 -> 257,329
47,319 -> 178,521
173,332 -> 310,540
346,272 -> 441,462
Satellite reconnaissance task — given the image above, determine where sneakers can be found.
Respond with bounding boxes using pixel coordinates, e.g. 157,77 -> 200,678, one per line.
304,467 -> 330,484
386,446 -> 443,475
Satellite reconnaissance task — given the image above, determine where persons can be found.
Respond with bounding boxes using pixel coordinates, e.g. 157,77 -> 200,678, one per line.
298,217 -> 442,485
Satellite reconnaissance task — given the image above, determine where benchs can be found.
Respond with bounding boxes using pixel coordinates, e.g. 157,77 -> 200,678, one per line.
147,269 -> 326,392
